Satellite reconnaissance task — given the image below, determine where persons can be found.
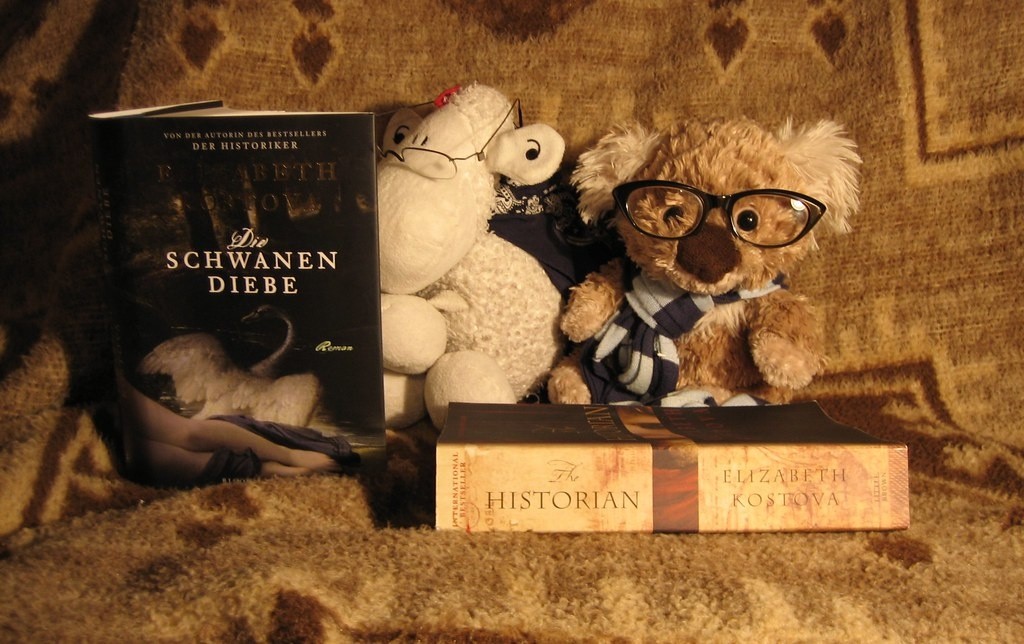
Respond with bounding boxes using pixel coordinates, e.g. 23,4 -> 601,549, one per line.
124,372 -> 337,479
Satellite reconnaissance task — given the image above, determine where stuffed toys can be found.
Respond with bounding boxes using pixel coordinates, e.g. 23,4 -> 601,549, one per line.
361,86 -> 861,431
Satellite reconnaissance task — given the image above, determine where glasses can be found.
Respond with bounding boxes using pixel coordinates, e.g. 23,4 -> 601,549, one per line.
612,179 -> 827,249
375,98 -> 523,178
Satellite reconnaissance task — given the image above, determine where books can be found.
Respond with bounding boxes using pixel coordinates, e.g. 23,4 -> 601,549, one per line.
86,101 -> 383,487
434,402 -> 908,530
